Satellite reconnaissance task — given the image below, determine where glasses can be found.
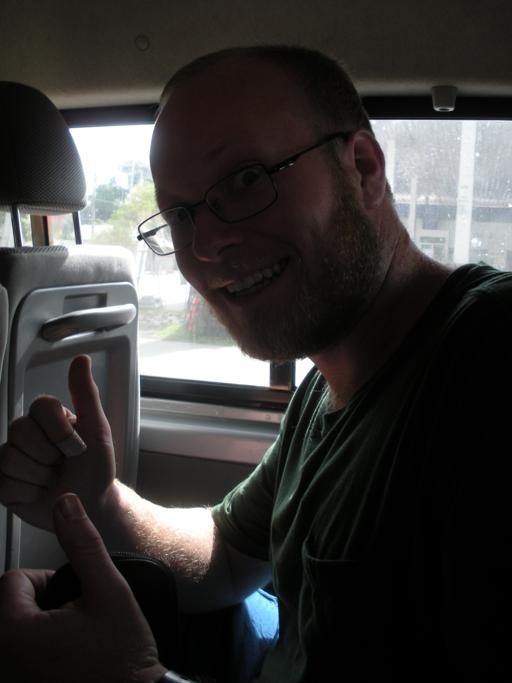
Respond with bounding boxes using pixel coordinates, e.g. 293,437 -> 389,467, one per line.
136,131 -> 347,258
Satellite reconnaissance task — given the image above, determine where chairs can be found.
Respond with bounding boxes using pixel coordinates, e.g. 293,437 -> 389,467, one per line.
0,77 -> 144,571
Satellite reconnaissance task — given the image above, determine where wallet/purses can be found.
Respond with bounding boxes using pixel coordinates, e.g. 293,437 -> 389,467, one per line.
36,549 -> 185,653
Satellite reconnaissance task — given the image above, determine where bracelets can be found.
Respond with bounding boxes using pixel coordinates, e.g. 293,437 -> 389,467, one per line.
159,669 -> 198,683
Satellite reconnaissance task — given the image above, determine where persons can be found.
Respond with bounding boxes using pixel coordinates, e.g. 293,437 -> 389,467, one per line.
1,43 -> 512,683
